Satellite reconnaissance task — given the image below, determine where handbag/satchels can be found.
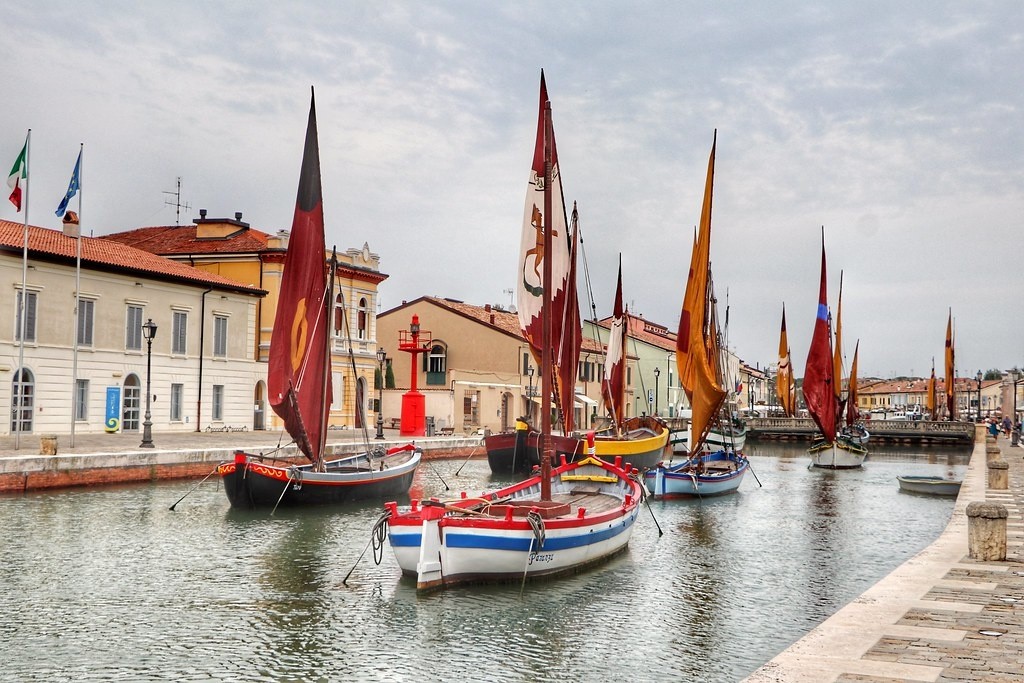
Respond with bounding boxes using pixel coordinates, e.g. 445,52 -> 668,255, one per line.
996,421 -> 1001,430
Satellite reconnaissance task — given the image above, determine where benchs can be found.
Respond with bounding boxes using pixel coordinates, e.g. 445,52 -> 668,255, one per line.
329,424 -> 348,430
440,428 -> 455,435
206,425 -> 248,432
390,418 -> 401,428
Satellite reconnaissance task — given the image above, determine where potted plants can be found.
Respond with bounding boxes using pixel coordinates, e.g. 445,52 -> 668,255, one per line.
591,413 -> 598,429
551,415 -> 556,433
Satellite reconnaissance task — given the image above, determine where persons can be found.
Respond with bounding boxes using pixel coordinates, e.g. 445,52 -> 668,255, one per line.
913,413 -> 918,420
982,414 -> 1012,439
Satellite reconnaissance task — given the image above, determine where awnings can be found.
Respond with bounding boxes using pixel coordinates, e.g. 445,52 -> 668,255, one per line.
528,395 -> 598,408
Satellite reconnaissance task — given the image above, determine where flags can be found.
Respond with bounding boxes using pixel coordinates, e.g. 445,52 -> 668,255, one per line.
7,139 -> 26,212
54,148 -> 79,217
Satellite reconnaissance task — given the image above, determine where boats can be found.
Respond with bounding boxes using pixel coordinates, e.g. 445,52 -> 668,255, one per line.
896,474 -> 961,496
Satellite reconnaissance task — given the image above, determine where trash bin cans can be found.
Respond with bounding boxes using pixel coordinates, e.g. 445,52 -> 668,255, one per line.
426,416 -> 434,431
427,423 -> 435,436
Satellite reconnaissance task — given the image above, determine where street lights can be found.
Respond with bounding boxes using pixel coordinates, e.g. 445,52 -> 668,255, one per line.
982,395 -> 1003,419
654,367 -> 660,416
967,382 -> 974,422
1010,368 -> 1020,447
750,380 -> 755,413
527,365 -> 534,427
977,370 -> 982,423
375,347 -> 387,439
138,318 -> 158,448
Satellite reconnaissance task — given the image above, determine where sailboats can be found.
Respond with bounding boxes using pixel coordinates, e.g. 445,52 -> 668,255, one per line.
643,128 -> 747,500
483,68 -> 671,477
801,226 -> 870,470
217,85 -> 422,510
385,99 -> 644,598
